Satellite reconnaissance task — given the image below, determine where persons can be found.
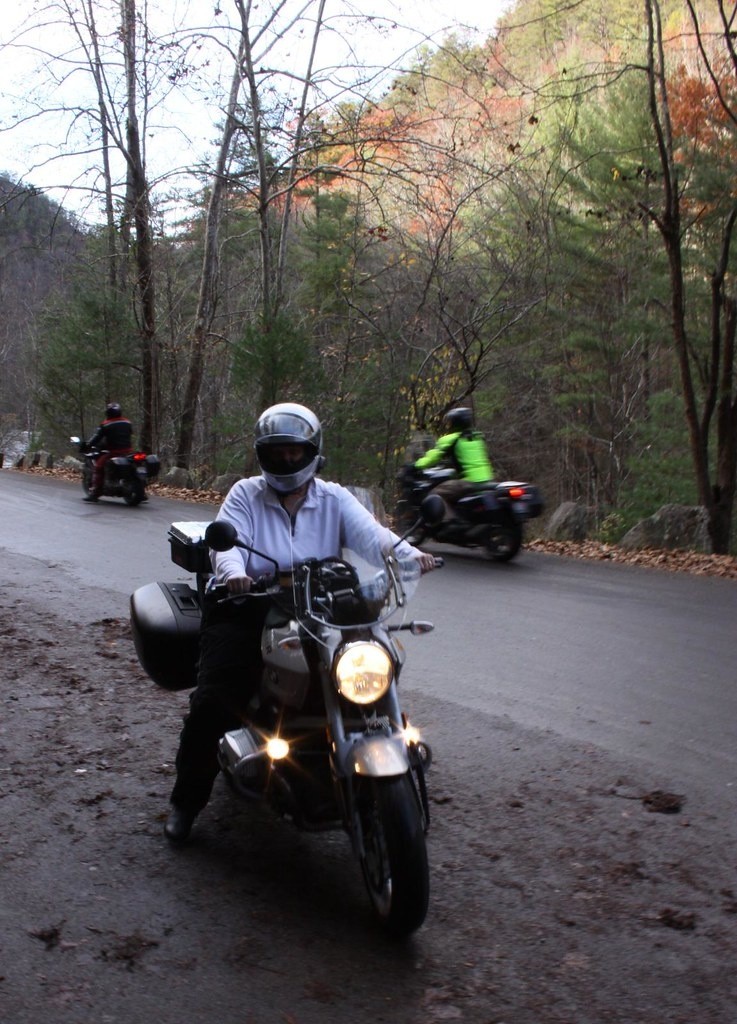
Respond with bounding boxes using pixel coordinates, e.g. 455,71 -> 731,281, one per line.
402,407 -> 493,531
164,401 -> 436,841
79,404 -> 149,501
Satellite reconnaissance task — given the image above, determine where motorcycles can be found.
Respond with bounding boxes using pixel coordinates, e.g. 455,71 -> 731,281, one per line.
70,432 -> 162,506
394,434 -> 545,561
127,492 -> 446,935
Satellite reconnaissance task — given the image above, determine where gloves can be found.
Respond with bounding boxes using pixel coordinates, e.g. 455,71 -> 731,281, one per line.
400,461 -> 416,471
78,441 -> 87,448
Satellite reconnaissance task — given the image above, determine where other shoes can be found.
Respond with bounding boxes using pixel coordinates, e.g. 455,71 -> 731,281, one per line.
83,497 -> 99,504
436,520 -> 468,539
165,805 -> 191,845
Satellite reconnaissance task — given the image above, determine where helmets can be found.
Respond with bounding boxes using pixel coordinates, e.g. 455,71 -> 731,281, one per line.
104,403 -> 122,420
253,402 -> 323,493
443,406 -> 474,434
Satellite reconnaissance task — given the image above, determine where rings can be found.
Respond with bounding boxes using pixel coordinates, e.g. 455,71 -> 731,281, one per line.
428,561 -> 432,565
